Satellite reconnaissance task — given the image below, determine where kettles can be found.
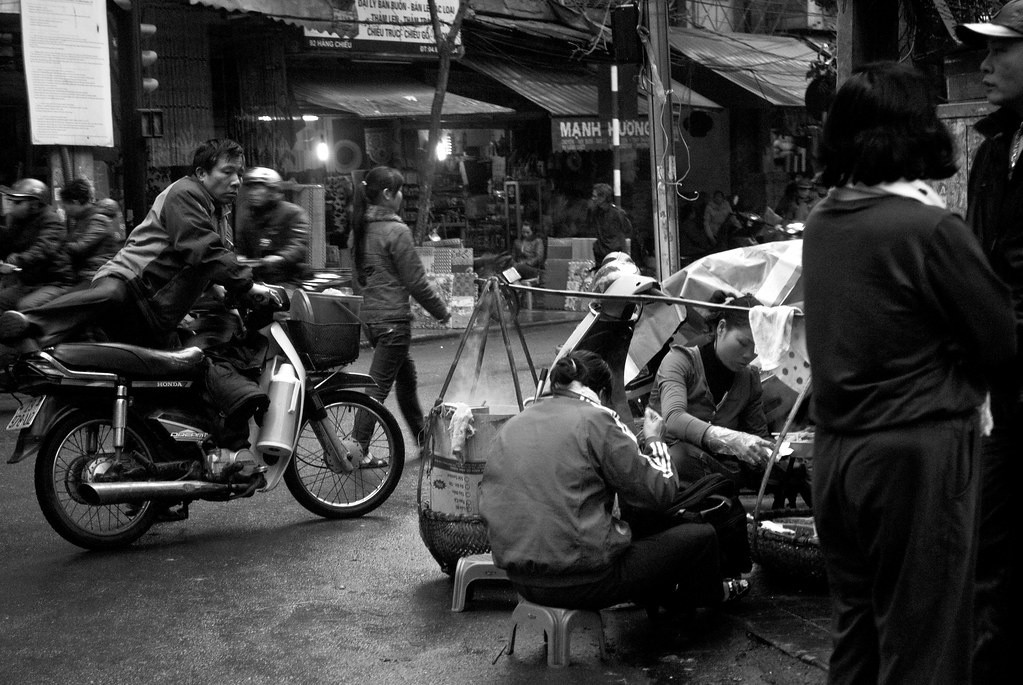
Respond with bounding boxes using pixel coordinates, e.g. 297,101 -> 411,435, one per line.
256,354 -> 301,457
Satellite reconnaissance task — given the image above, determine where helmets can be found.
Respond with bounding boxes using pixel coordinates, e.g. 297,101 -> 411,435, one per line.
243,167 -> 283,191
7,178 -> 49,206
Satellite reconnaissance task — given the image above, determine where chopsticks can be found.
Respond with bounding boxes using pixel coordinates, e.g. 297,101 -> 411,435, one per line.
636,415 -> 658,439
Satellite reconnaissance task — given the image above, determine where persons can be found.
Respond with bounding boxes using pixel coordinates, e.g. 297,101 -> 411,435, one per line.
348,166 -> 452,470
703,190 -> 759,252
6,177 -> 75,376
954,0 -> 1023,684
478,349 -> 750,634
645,293 -> 816,578
47,179 -> 127,346
784,177 -> 823,241
0,137 -> 284,513
587,183 -> 633,272
505,219 -> 545,280
234,166 -> 314,284
802,58 -> 1023,685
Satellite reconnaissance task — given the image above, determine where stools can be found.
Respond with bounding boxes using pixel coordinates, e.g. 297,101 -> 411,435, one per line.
522,273 -> 540,311
506,596 -> 608,668
450,553 -> 525,612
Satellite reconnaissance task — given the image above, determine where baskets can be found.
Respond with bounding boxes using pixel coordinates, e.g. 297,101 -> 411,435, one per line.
284,319 -> 361,363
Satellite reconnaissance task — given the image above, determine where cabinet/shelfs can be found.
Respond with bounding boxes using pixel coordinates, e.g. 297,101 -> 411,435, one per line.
400,168 -> 525,257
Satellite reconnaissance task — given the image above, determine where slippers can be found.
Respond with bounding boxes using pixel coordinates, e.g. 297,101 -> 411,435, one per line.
354,453 -> 388,468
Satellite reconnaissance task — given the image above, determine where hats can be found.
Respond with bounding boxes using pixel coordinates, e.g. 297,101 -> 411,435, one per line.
960,0 -> 1023,38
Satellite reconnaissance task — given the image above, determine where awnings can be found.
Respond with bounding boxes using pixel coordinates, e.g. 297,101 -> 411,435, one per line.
289,70 -> 517,122
669,24 -> 838,106
456,52 -> 724,153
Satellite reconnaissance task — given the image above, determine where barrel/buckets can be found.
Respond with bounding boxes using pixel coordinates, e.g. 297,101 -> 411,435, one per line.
289,288 -> 369,359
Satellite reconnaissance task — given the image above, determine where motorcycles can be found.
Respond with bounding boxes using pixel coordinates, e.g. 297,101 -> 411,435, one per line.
0,239 -> 406,550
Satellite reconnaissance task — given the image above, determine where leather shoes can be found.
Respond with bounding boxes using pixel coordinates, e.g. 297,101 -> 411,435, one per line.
725,578 -> 750,602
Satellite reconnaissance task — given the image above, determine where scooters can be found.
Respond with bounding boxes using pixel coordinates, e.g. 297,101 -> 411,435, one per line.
546,234 -> 814,506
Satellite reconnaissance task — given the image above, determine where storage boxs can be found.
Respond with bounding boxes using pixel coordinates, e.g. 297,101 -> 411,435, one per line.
427,455 -> 487,515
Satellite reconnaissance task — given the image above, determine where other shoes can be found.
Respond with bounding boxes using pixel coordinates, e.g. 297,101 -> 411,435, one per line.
0,310 -> 27,361
